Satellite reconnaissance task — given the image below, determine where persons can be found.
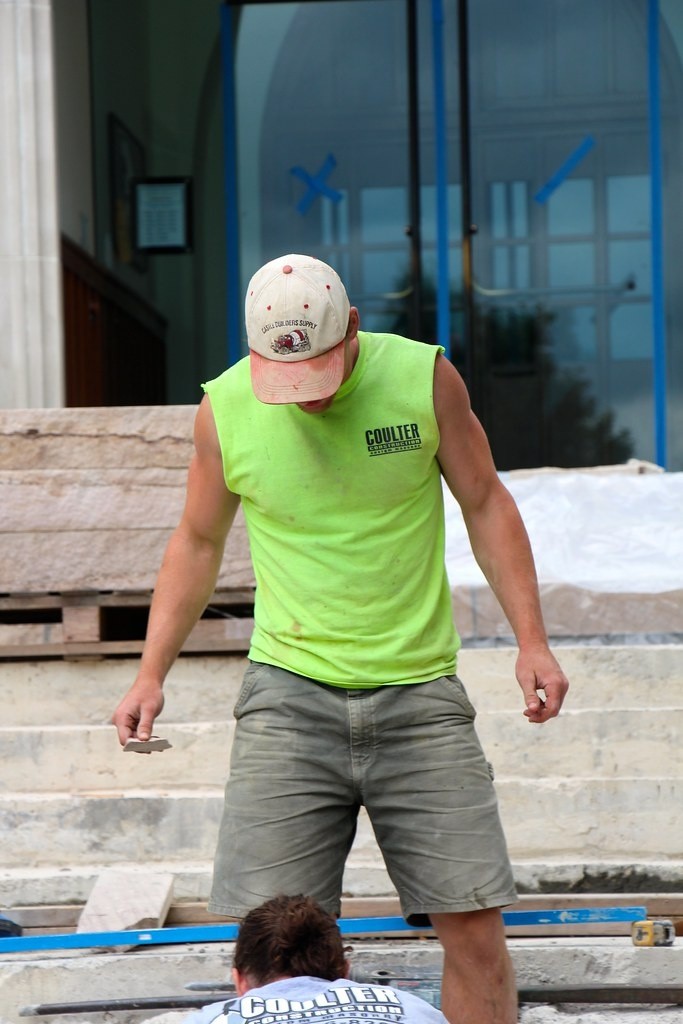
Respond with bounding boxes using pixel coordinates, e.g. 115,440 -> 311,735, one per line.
112,255 -> 570,1024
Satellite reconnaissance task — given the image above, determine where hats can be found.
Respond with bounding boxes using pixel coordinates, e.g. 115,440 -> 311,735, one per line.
245,253 -> 352,403
